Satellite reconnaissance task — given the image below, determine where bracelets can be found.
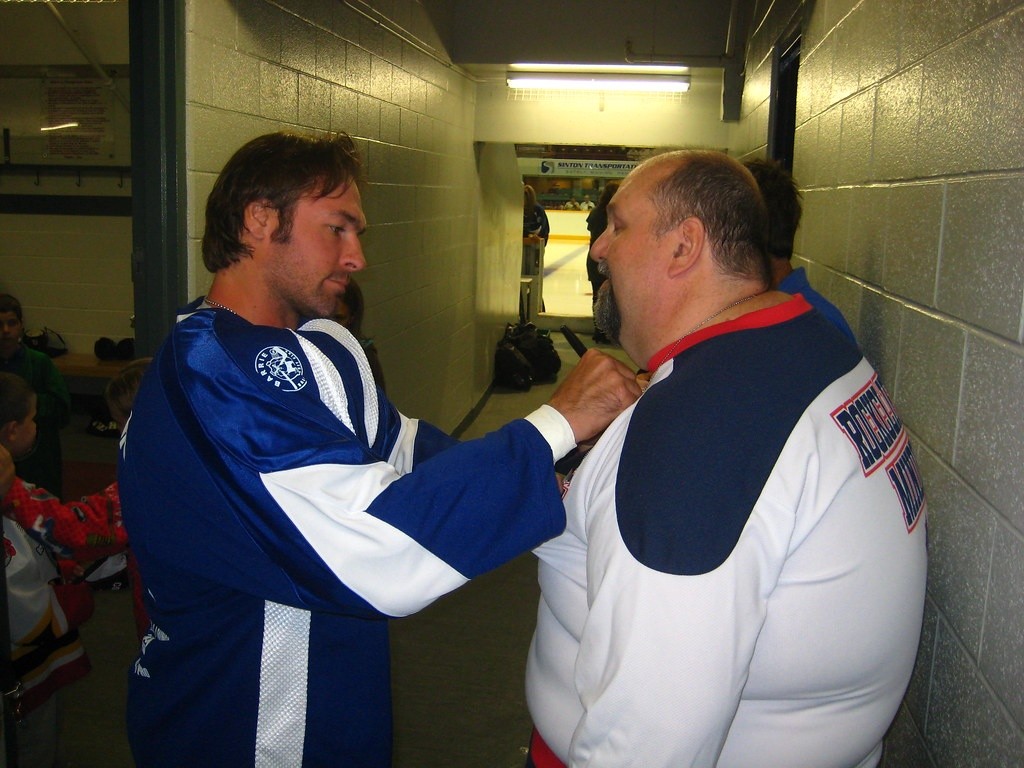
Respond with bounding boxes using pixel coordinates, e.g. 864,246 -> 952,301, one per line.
574,205 -> 576,206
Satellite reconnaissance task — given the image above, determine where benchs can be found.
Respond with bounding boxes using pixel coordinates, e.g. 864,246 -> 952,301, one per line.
51,353 -> 124,380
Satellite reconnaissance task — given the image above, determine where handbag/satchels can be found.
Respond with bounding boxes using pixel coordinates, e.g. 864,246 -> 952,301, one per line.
497,322 -> 561,389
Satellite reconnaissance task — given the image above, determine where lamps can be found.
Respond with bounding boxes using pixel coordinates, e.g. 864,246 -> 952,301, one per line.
502,70 -> 692,91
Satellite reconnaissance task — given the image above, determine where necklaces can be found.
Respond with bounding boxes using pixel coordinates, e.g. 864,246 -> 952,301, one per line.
204,297 -> 237,315
641,295 -> 752,395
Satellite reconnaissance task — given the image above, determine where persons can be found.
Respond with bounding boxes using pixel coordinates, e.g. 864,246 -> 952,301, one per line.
524,185 -> 549,245
743,159 -> 857,343
335,279 -> 385,388
580,195 -> 595,210
565,194 -> 580,211
122,133 -> 651,768
0,294 -> 154,646
586,181 -> 618,344
525,152 -> 925,768
